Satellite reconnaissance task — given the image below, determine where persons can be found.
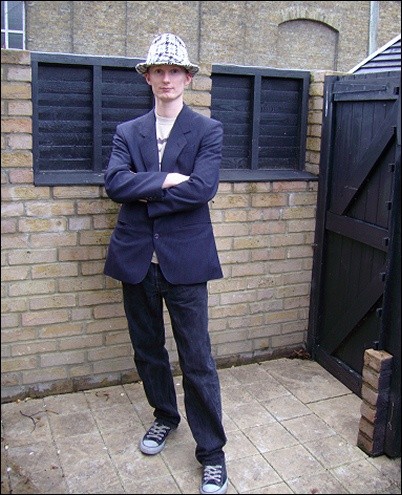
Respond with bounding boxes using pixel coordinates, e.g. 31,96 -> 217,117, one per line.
103,32 -> 229,495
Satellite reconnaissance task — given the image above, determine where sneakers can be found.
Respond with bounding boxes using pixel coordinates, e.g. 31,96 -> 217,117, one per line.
200,456 -> 227,495
139,419 -> 171,455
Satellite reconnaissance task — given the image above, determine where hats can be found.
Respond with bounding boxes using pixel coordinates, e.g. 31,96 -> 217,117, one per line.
134,33 -> 199,77
